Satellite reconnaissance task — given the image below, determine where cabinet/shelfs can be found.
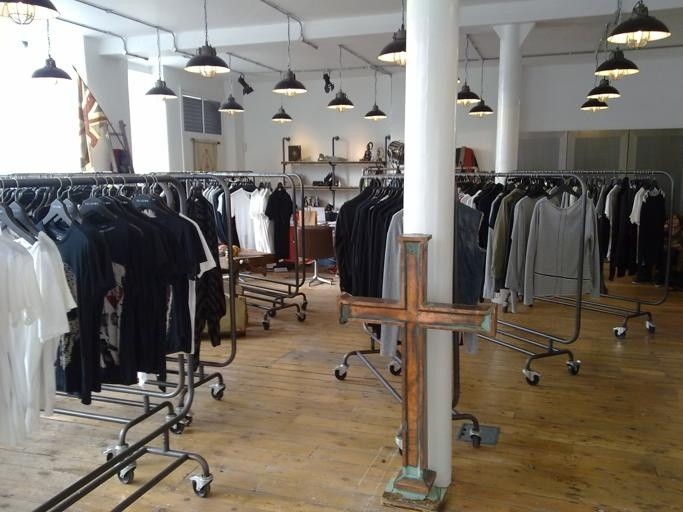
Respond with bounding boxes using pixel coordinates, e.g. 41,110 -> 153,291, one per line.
280,160 -> 385,227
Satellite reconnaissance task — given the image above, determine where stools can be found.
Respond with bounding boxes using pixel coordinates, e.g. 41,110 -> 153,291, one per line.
295,226 -> 336,287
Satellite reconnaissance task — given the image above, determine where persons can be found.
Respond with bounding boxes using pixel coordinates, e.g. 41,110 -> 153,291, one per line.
655,211 -> 682,292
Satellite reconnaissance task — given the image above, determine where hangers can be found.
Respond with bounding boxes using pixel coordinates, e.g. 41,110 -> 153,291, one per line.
360,165 -> 402,213
0,170 -> 284,246
452,168 -> 663,204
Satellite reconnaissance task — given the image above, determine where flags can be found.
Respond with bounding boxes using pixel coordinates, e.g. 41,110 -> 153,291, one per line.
77,75 -> 110,171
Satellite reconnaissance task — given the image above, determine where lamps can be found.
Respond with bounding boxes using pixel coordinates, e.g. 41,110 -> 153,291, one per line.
459,34 -> 494,117
577,0 -> 673,113
